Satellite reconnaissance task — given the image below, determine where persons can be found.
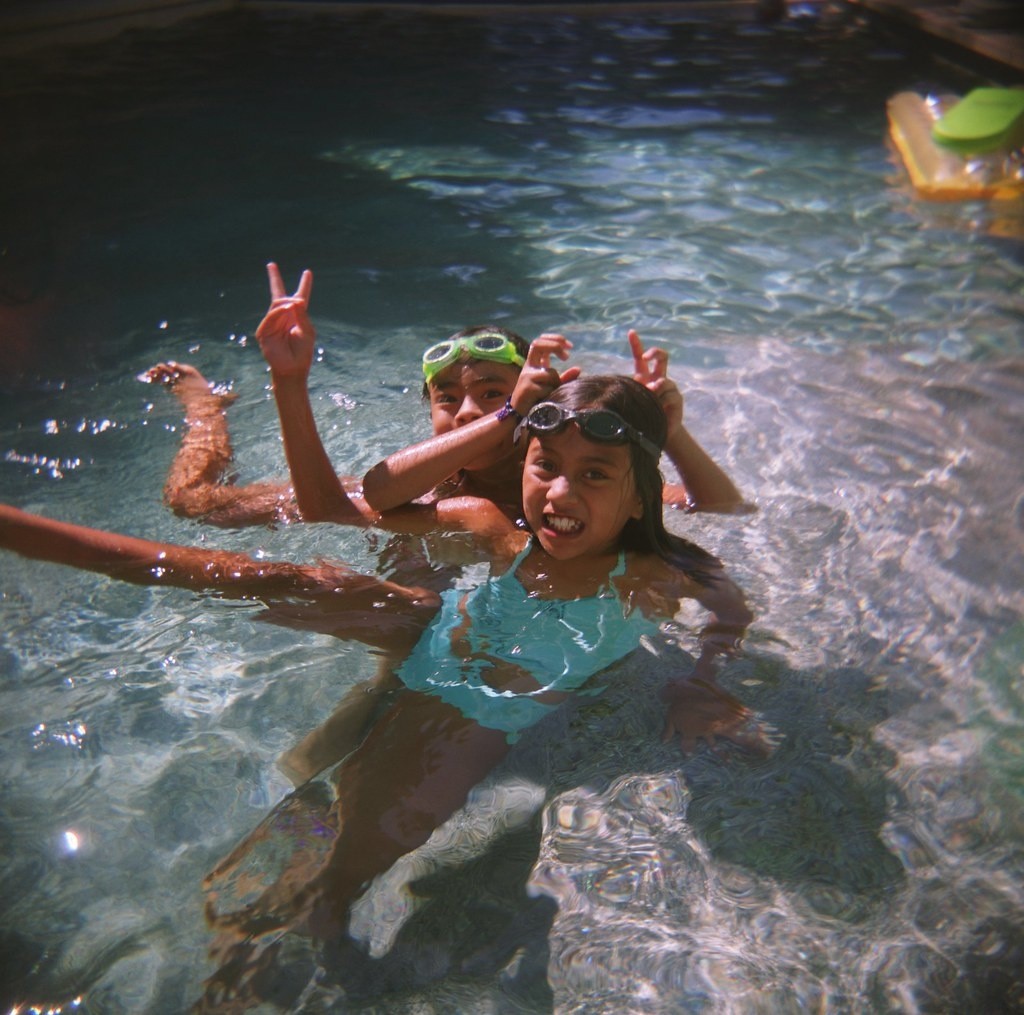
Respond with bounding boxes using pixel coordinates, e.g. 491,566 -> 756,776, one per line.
0,262 -> 769,1015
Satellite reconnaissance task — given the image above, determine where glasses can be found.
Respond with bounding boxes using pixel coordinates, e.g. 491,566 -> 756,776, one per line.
423,327 -> 518,393
527,401 -> 634,443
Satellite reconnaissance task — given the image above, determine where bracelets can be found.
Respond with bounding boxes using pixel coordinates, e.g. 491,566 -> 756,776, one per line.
496,396 -> 524,425
